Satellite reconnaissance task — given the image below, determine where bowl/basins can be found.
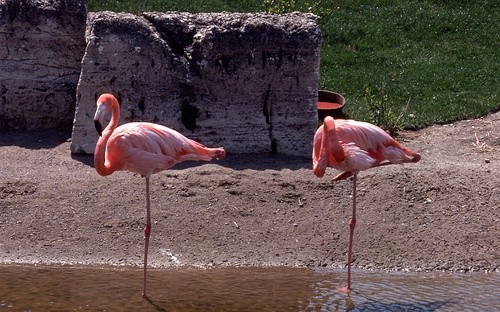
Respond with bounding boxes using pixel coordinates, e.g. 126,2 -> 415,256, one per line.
317,89 -> 345,115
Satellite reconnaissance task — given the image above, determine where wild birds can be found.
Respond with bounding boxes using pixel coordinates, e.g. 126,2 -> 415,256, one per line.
94,93 -> 226,302
312,115 -> 421,293
